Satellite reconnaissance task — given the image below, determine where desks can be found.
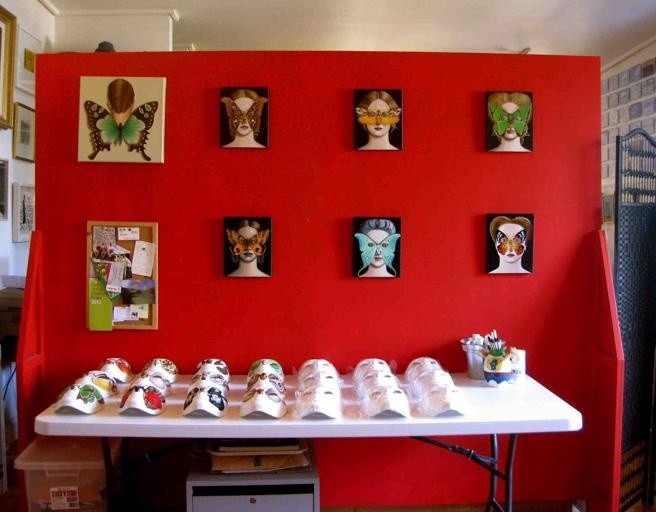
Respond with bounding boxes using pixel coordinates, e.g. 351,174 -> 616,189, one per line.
0,287 -> 24,337
34,371 -> 583,512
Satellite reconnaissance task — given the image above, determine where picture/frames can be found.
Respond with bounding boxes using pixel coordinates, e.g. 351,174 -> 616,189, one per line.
0,5 -> 17,130
12,182 -> 35,243
0,159 -> 9,220
12,102 -> 36,162
86,221 -> 159,332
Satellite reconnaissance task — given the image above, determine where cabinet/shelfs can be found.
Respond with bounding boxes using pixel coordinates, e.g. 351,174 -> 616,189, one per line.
186,455 -> 319,512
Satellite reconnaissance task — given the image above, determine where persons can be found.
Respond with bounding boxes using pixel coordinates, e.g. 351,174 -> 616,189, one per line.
352,217 -> 400,277
485,215 -> 532,273
225,219 -> 272,277
485,92 -> 533,152
353,90 -> 402,150
218,88 -> 268,147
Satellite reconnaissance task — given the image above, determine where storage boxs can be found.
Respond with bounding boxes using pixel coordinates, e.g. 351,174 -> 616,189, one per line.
14,435 -> 123,511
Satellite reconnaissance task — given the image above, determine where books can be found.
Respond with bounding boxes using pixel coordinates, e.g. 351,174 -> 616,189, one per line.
204,436 -> 312,477
